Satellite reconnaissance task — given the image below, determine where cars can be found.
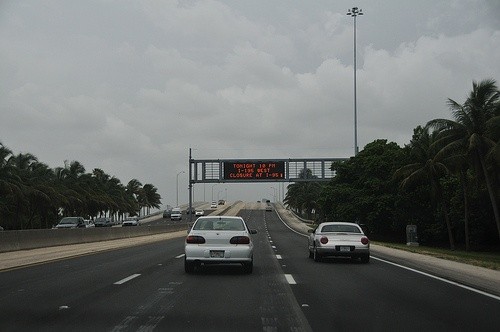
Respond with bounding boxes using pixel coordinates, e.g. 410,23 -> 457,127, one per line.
123,216 -> 139,226
307,222 -> 370,263
162,199 -> 226,221
184,216 -> 258,274
94,218 -> 112,227
265,206 -> 273,212
84,219 -> 95,228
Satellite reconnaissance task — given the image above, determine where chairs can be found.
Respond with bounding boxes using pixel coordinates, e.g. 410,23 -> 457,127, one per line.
204,224 -> 213,230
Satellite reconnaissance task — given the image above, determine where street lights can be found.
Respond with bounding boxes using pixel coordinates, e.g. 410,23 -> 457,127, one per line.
345,5 -> 365,155
193,173 -> 200,203
177,171 -> 185,207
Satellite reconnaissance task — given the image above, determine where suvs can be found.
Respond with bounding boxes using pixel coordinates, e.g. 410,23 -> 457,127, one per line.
56,217 -> 86,229
